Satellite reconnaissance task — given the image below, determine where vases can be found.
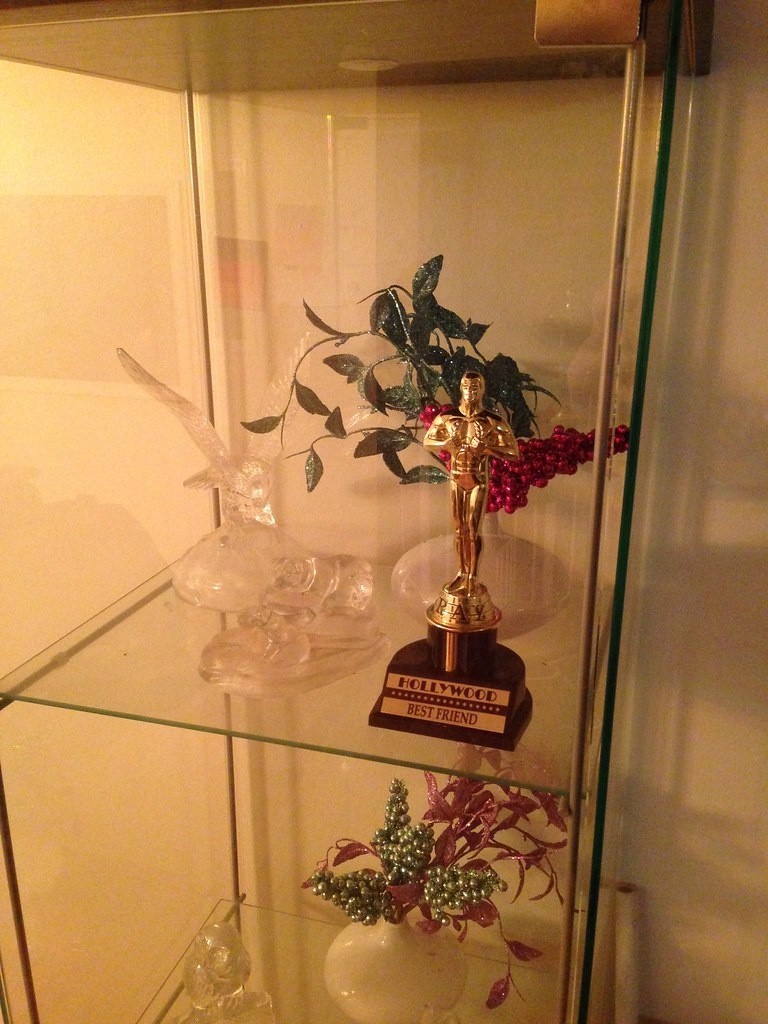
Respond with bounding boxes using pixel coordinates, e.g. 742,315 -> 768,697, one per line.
324,914 -> 469,1024
390,511 -> 570,643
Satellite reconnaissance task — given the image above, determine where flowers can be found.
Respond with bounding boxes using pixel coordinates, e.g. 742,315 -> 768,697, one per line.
239,255 -> 628,514
301,741 -> 580,1009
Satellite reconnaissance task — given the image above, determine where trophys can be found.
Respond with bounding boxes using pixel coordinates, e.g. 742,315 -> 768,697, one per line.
368,372 -> 533,751
